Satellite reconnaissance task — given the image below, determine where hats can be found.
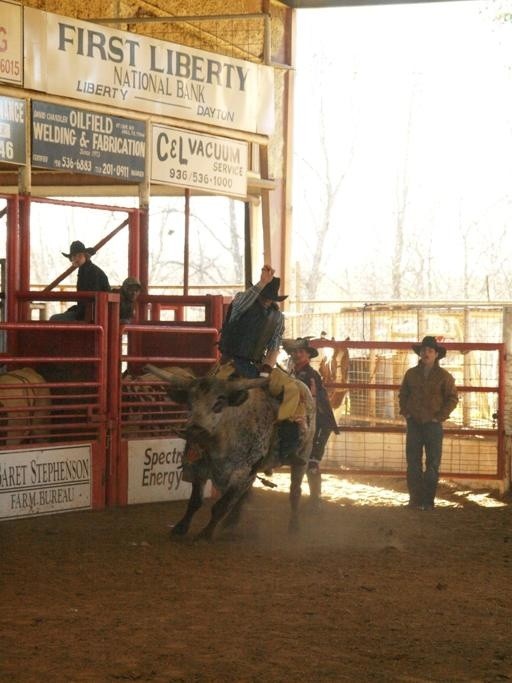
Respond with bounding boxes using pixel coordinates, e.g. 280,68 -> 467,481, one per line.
123,277 -> 142,289
411,336 -> 446,358
247,276 -> 288,302
282,338 -> 319,359
62,241 -> 96,259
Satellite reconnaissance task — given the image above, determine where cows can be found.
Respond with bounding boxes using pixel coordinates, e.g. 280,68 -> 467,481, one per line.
121,367 -> 196,437
0,366 -> 52,445
144,363 -> 317,547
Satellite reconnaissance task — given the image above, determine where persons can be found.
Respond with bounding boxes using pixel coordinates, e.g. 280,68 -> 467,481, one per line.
116,275 -> 141,324
49,240 -> 109,324
279,336 -> 340,531
219,263 -> 303,469
398,336 -> 459,512
